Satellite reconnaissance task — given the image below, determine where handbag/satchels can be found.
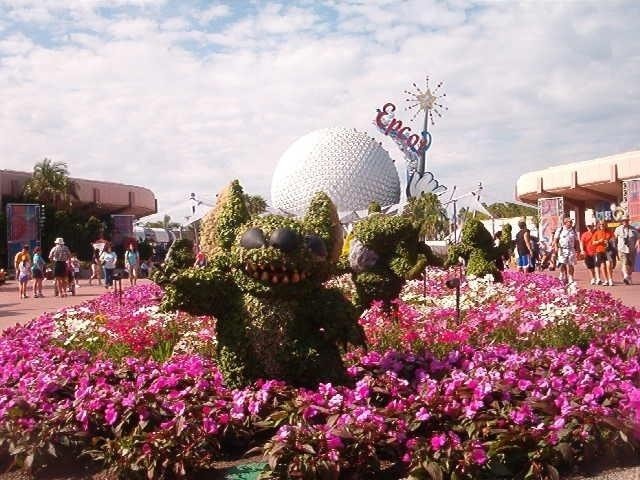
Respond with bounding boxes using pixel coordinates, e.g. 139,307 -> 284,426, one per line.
527,265 -> 535,272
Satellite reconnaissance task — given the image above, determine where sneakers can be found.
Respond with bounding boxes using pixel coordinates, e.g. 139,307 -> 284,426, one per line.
75,284 -> 80,287
591,278 -> 633,286
568,281 -> 577,286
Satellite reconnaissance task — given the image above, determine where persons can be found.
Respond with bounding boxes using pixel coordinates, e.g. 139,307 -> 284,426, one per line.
12,236 -> 154,301
194,252 -> 207,269
492,214 -> 640,286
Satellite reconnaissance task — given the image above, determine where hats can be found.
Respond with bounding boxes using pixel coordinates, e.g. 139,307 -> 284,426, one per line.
620,216 -> 629,221
22,255 -> 28,261
54,238 -> 65,245
586,221 -> 594,226
564,218 -> 574,223
